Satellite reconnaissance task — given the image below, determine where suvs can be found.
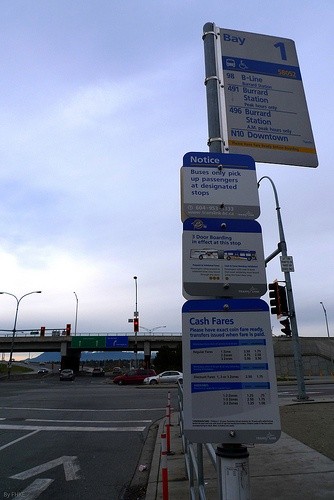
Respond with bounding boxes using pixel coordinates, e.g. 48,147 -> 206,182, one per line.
92,368 -> 105,377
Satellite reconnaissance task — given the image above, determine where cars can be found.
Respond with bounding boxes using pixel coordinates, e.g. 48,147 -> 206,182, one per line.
24,361 -> 31,364
143,370 -> 184,384
38,368 -> 48,374
114,367 -> 122,374
112,368 -> 157,385
59,369 -> 75,381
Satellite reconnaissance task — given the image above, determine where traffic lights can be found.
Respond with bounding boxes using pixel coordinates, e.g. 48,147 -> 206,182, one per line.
66,323 -> 72,336
280,317 -> 291,336
268,279 -> 282,315
134,318 -> 139,332
40,326 -> 46,337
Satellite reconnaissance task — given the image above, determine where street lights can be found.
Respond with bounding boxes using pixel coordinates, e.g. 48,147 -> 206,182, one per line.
73,291 -> 78,335
133,276 -> 140,371
320,301 -> 329,337
0,291 -> 42,379
